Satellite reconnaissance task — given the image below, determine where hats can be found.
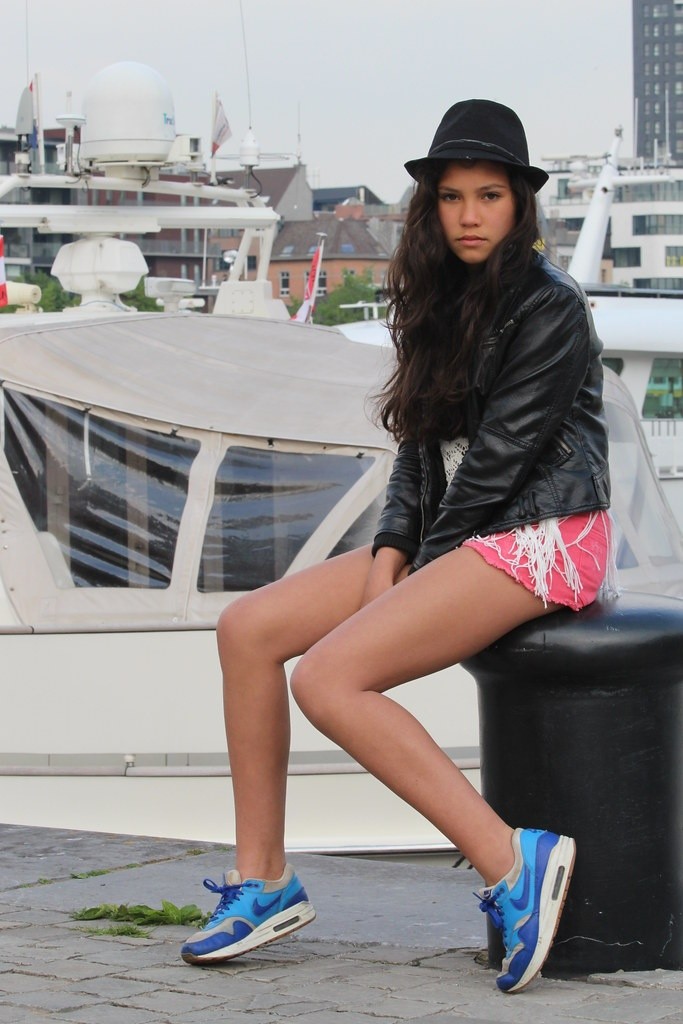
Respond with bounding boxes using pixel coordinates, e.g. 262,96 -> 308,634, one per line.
403,99 -> 549,194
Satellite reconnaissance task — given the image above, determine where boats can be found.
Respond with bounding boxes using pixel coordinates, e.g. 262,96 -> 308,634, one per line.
1,69 -> 292,323
0,311 -> 683,858
330,124 -> 683,563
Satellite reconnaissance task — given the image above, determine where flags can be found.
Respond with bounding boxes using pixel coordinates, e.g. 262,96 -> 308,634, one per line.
30,71 -> 46,166
211,90 -> 232,157
289,244 -> 323,322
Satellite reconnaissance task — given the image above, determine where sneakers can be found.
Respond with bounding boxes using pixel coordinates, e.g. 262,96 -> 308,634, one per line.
181,862 -> 316,966
471,828 -> 577,994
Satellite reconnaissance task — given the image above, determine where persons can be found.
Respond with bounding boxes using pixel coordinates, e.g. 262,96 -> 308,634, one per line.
180,100 -> 611,995
